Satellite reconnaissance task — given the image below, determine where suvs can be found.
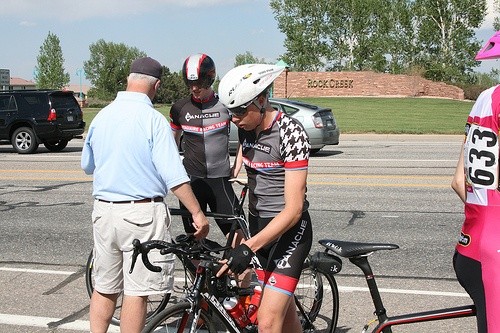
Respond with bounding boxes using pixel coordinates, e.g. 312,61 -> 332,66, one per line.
0,88 -> 86,154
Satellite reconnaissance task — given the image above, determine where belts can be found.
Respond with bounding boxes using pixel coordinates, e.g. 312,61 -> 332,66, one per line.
95,196 -> 163,205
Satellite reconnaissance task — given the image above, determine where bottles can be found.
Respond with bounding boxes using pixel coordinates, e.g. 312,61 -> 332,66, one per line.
247,286 -> 262,326
223,296 -> 250,328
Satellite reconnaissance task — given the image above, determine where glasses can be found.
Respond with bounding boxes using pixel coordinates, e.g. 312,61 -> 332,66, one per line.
227,97 -> 260,118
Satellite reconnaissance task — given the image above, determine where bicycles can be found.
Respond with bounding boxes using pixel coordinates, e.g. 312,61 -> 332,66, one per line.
85,177 -> 478,333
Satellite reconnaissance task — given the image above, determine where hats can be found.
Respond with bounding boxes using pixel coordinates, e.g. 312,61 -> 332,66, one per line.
130,56 -> 163,79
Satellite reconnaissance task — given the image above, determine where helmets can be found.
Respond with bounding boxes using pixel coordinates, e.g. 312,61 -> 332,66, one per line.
475,30 -> 500,60
181,54 -> 216,88
218,63 -> 285,109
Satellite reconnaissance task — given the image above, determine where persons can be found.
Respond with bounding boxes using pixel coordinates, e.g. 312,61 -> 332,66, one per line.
218,63 -> 312,333
168,52 -> 243,328
81,56 -> 209,333
452,31 -> 500,333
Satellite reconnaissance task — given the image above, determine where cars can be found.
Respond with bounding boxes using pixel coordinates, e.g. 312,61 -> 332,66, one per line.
228,98 -> 339,157
